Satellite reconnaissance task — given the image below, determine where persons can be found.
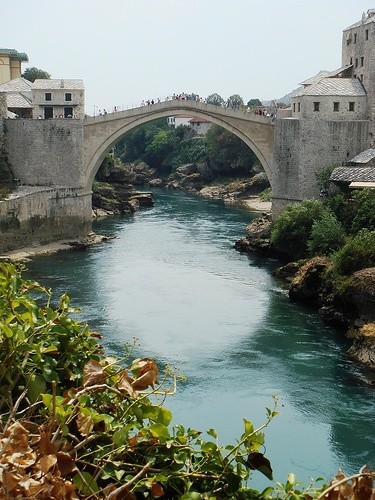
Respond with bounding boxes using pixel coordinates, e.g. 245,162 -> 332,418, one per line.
30,92 -> 277,122
